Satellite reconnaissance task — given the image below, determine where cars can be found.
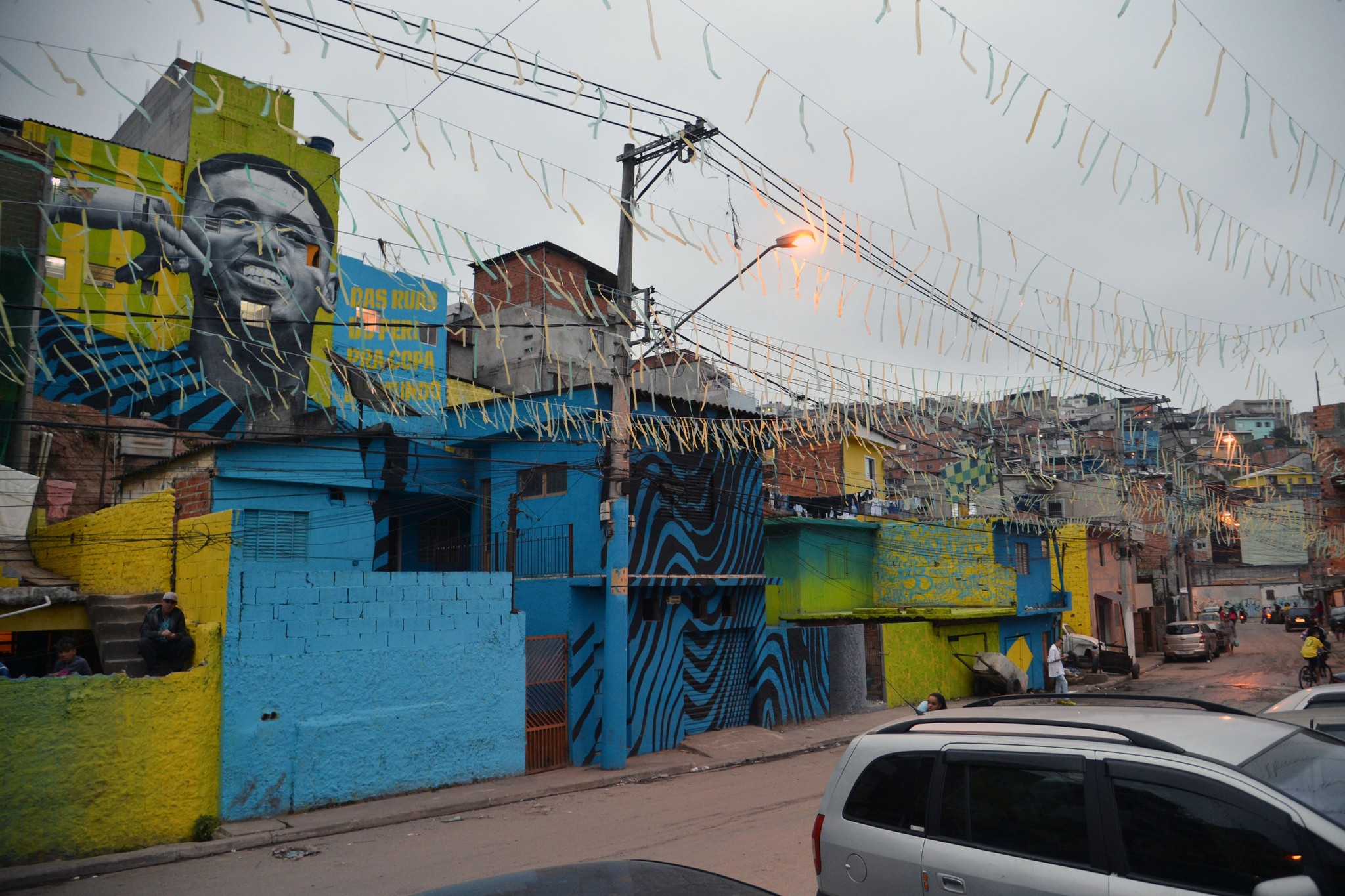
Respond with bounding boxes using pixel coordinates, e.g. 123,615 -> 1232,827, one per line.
398,858 -> 782,896
1060,622 -> 1108,663
1255,670 -> 1345,744
1196,606 -> 1238,653
1327,605 -> 1345,631
1284,607 -> 1320,631
1162,621 -> 1219,661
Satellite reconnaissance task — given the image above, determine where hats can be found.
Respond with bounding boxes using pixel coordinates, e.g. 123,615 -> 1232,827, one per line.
1223,614 -> 1228,617
164,592 -> 178,601
1268,605 -> 1271,606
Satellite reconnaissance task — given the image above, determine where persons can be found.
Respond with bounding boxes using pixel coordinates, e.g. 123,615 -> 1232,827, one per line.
1307,621 -> 1330,652
1301,630 -> 1332,686
1047,637 -> 1076,706
1260,602 -> 1300,624
138,591 -> 194,678
915,692 -> 947,713
1229,607 -> 1237,626
1239,605 -> 1248,621
1219,615 -> 1237,656
1313,598 -> 1324,628
1218,606 -> 1228,621
46,637 -> 91,678
34,153 -> 366,441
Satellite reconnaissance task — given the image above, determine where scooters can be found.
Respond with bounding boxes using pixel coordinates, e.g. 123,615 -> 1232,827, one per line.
1265,613 -> 1275,624
1239,614 -> 1247,624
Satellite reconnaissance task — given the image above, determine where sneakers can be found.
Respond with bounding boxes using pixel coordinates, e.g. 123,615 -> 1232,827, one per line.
1056,699 -> 1064,704
1064,699 -> 1075,705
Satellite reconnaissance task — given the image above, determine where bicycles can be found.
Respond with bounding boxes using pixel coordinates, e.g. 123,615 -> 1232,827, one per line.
1298,651 -> 1334,691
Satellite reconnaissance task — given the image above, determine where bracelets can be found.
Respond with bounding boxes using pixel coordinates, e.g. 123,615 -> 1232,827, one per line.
1057,658 -> 1060,662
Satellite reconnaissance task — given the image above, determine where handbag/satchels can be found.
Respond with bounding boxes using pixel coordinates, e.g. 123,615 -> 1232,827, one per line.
1233,638 -> 1239,647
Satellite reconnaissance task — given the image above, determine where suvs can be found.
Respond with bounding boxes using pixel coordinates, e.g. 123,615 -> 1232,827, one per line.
808,690 -> 1345,896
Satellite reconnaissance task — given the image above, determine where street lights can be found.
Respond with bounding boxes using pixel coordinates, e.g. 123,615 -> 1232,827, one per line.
600,229 -> 821,772
